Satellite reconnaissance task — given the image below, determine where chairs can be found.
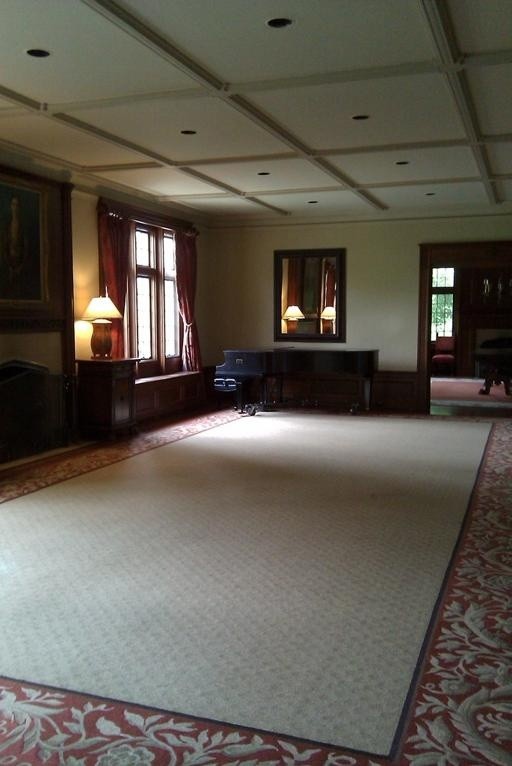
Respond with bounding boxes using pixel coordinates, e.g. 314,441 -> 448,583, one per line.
431,332 -> 459,378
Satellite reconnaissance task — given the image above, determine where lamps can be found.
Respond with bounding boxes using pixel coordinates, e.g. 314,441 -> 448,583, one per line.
80,297 -> 124,361
283,305 -> 306,334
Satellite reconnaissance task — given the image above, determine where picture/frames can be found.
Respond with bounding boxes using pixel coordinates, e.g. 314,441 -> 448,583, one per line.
0,163 -> 76,333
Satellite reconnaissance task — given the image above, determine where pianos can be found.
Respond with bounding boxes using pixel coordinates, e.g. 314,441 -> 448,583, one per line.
214,347 -> 295,410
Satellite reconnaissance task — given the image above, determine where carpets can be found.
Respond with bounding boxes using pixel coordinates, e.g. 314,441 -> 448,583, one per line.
1,405 -> 511,766
430,376 -> 511,409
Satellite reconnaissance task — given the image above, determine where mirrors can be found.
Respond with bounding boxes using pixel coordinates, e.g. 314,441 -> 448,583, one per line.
273,247 -> 346,343
